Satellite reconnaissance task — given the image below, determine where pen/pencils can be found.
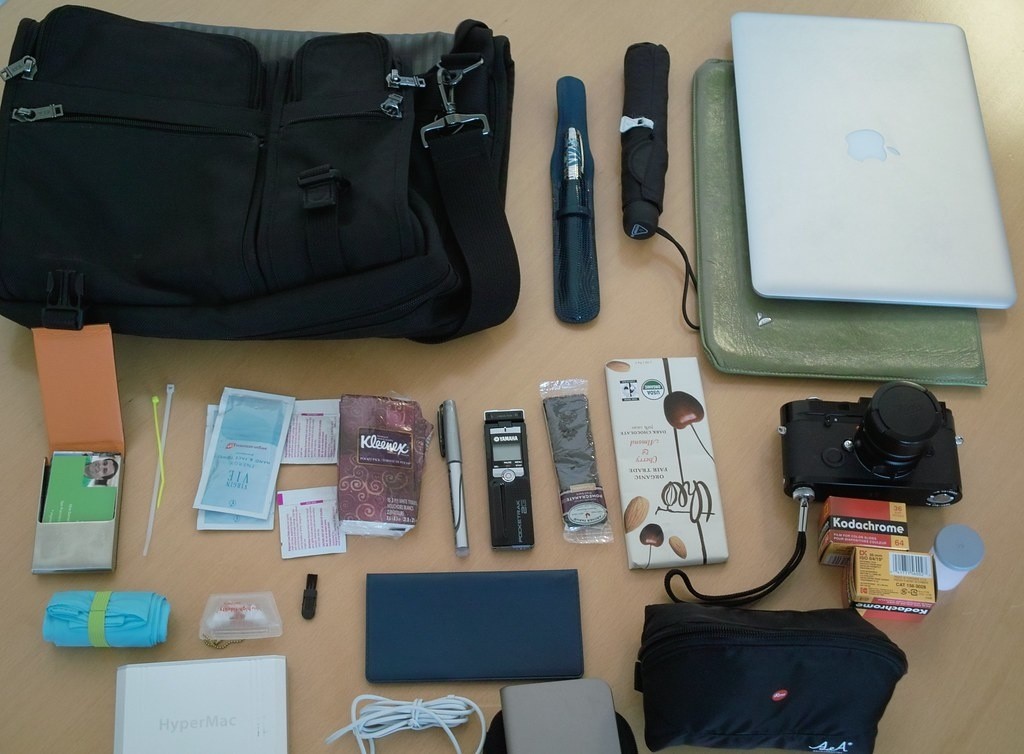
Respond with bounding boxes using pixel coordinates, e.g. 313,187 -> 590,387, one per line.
433,398 -> 470,558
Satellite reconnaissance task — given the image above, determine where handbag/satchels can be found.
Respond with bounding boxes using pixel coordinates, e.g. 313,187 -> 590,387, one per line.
0,5 -> 524,349
633,602 -> 909,754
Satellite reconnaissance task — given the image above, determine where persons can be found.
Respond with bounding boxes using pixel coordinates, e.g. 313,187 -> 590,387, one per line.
84,455 -> 118,487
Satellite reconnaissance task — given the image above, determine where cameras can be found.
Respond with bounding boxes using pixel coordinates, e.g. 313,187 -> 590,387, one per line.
778,380 -> 962,506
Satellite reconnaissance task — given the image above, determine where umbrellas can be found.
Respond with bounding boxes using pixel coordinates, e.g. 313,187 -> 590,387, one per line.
617,42 -> 701,332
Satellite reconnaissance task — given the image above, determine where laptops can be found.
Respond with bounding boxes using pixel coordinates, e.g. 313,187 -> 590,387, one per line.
731,12 -> 1017,310
